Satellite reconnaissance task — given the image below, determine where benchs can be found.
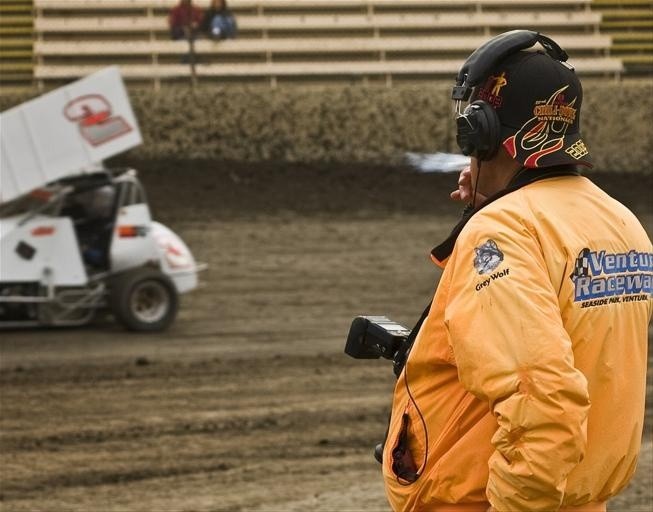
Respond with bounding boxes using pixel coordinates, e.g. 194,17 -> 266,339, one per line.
31,0 -> 625,95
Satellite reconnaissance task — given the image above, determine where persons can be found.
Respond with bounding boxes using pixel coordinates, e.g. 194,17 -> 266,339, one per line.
375,23 -> 652,512
198,0 -> 237,65
169,0 -> 204,63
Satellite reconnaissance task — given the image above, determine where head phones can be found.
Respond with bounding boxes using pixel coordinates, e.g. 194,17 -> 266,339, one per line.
451,29 -> 577,161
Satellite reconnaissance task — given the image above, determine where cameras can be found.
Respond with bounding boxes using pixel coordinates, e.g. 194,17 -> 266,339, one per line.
344,315 -> 416,464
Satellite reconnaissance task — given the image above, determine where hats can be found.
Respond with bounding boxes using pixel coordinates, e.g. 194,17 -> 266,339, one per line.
471,50 -> 594,169
